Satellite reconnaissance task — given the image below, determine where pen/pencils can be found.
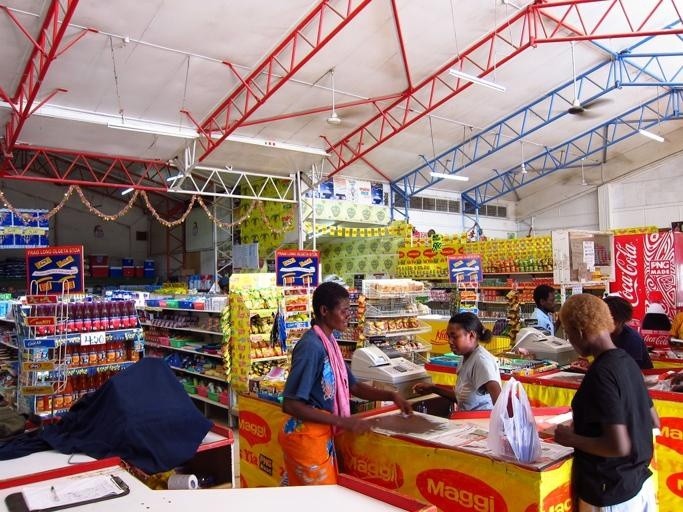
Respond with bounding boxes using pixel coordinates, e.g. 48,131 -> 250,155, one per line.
51,486 -> 59,501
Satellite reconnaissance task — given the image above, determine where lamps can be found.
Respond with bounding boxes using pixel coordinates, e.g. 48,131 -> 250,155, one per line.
429,116 -> 470,182
447,0 -> 506,95
107,35 -> 195,142
638,73 -> 665,144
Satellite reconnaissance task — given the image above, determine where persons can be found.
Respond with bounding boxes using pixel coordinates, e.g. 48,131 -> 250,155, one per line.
553,293 -> 659,512
7,287 -> 18,298
413,312 -> 503,410
529,285 -> 561,336
603,296 -> 653,369
278,282 -> 414,486
669,312 -> 683,345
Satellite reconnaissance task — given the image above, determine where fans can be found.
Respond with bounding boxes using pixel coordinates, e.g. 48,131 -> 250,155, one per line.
579,158 -> 588,187
567,39 -> 586,115
326,66 -> 341,127
519,140 -> 528,175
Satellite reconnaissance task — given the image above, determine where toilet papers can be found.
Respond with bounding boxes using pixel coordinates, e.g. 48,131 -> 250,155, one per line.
169,475 -> 196,488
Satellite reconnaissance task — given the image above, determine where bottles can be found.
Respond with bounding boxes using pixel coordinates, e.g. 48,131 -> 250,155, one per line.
338,345 -> 354,359
332,324 -> 356,340
65,343 -> 138,368
430,302 -> 449,317
345,287 -> 362,302
35,371 -> 119,410
33,300 -> 138,334
348,306 -> 360,322
640,290 -> 671,348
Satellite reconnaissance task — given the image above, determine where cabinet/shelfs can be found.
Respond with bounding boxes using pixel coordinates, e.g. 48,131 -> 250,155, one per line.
134,306 -> 232,430
0,297 -> 20,439
360,278 -> 434,365
563,281 -> 607,304
332,292 -> 364,363
433,273 -> 562,341
227,288 -> 315,429
16,301 -> 145,430
